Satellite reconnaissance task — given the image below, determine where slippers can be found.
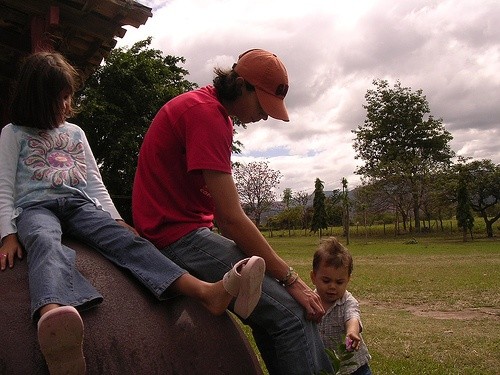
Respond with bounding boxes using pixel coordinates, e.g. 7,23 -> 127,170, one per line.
38,305 -> 86,375
223,256 -> 266,319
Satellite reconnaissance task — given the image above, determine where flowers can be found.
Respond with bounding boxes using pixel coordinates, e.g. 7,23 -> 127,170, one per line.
318,333 -> 359,375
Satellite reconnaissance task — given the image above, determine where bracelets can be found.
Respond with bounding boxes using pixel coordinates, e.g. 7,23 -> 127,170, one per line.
275,267 -> 299,288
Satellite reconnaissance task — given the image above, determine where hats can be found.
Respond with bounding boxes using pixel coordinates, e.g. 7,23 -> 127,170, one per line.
232,48 -> 289,122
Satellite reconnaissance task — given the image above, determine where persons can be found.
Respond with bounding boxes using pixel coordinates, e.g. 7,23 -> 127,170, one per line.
306,237 -> 372,375
132,49 -> 335,375
1,52 -> 266,375
